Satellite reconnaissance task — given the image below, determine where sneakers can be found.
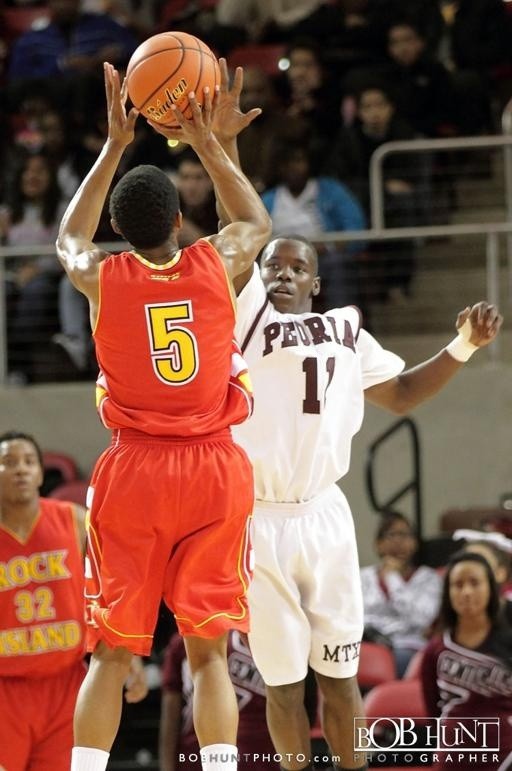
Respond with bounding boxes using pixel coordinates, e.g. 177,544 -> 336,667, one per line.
51,330 -> 90,379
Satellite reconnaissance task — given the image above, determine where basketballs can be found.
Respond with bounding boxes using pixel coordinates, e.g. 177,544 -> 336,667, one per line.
125,30 -> 221,126
